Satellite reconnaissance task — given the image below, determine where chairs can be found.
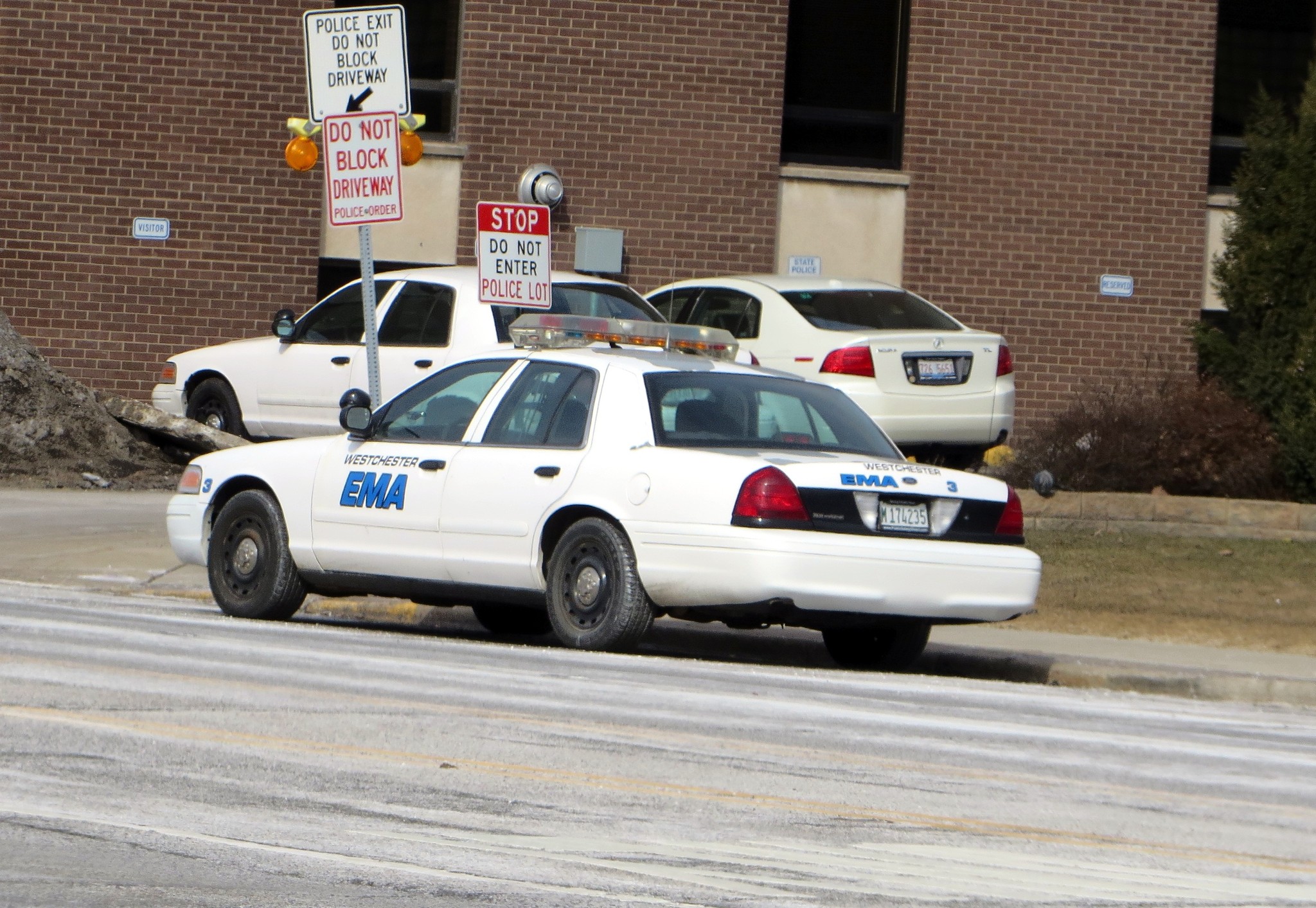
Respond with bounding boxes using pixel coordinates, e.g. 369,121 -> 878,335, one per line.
534,400 -> 589,447
423,396 -> 478,442
674,399 -> 729,448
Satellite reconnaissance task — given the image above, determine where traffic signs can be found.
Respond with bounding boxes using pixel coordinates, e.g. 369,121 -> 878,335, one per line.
301,3 -> 412,127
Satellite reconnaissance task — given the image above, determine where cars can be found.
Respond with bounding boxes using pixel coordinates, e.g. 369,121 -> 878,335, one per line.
146,260 -> 741,446
161,308 -> 1044,679
631,271 -> 1016,470
